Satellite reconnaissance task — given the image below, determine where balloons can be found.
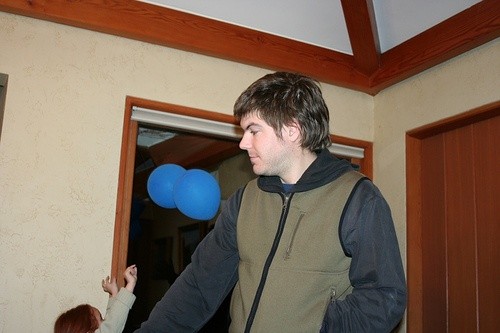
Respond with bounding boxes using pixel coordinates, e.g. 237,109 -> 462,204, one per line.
173,170 -> 221,220
146,164 -> 186,209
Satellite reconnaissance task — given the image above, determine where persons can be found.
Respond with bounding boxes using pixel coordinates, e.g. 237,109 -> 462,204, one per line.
138,71 -> 407,333
54,264 -> 137,333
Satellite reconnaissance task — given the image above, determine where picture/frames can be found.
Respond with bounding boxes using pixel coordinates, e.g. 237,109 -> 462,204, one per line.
176,221 -> 207,275
